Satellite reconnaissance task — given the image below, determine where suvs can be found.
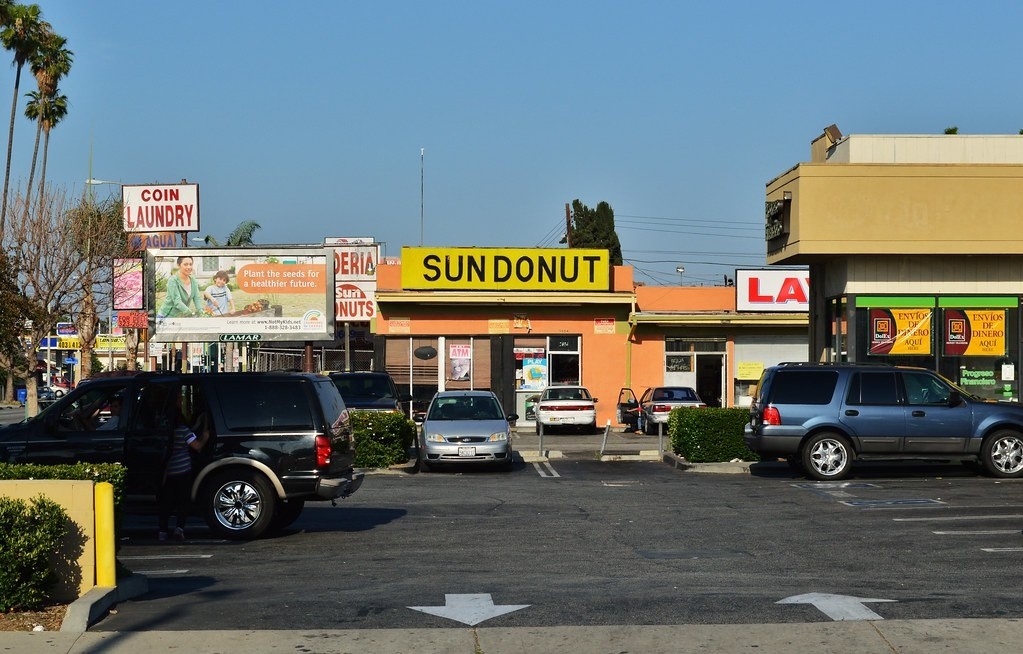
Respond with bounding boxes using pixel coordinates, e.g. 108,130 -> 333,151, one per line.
740,359 -> 1022,483
327,370 -> 405,420
0,369 -> 365,545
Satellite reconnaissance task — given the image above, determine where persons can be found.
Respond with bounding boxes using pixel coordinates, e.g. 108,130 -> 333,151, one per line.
203,270 -> 235,315
158,256 -> 201,317
627,404 -> 643,435
158,411 -> 209,541
77,396 -> 123,431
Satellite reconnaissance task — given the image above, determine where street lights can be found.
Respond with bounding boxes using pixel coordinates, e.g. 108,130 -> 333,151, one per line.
676,266 -> 685,285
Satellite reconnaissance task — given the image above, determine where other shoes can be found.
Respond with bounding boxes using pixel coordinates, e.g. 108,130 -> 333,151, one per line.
174,527 -> 187,541
635,430 -> 642,434
159,532 -> 167,541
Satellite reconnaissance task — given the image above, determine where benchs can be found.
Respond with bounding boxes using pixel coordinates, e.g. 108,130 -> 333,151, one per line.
443,400 -> 491,417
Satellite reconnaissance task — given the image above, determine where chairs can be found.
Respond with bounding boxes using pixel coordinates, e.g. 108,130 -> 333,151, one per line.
549,392 -> 558,398
573,393 -> 582,399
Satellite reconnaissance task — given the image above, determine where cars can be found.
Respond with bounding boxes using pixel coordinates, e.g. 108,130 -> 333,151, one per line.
411,390 -> 520,474
532,385 -> 598,435
41,381 -> 70,398
39,386 -> 56,401
616,387 -> 708,435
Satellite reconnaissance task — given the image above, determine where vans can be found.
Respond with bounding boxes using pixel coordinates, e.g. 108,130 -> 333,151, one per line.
44,375 -> 70,388
